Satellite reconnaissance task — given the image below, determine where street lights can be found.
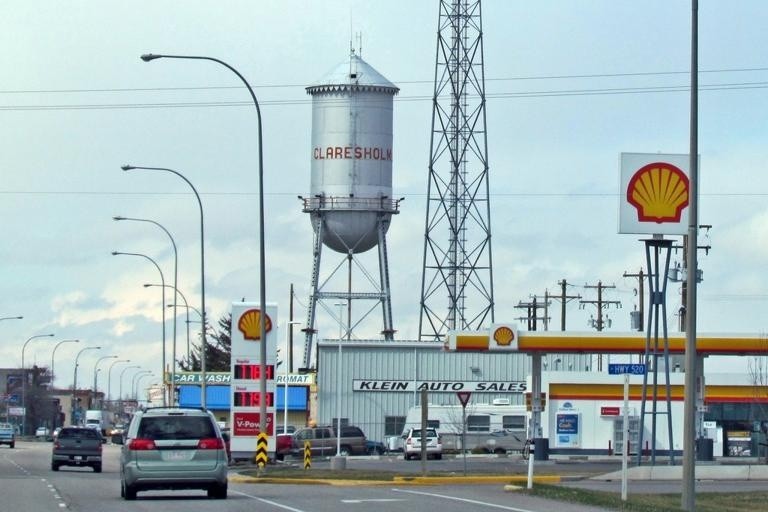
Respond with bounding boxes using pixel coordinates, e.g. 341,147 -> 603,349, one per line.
0,314 -> 158,437
137,50 -> 270,471
110,159 -> 214,415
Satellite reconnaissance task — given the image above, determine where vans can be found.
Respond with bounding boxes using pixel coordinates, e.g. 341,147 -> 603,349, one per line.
45,426 -> 108,473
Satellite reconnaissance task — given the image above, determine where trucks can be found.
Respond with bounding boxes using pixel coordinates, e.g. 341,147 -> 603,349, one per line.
0,422 -> 16,449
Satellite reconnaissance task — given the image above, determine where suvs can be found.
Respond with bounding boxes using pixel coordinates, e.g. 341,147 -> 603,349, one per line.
399,426 -> 444,460
107,404 -> 233,502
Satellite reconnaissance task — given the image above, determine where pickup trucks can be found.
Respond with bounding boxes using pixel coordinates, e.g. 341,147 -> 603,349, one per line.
289,424 -> 367,460
219,422 -> 292,462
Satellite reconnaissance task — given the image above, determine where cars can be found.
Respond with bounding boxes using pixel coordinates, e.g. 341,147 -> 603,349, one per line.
276,424 -> 296,434
366,439 -> 387,456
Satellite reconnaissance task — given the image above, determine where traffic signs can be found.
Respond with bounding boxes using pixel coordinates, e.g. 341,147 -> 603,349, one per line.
608,363 -> 646,375
